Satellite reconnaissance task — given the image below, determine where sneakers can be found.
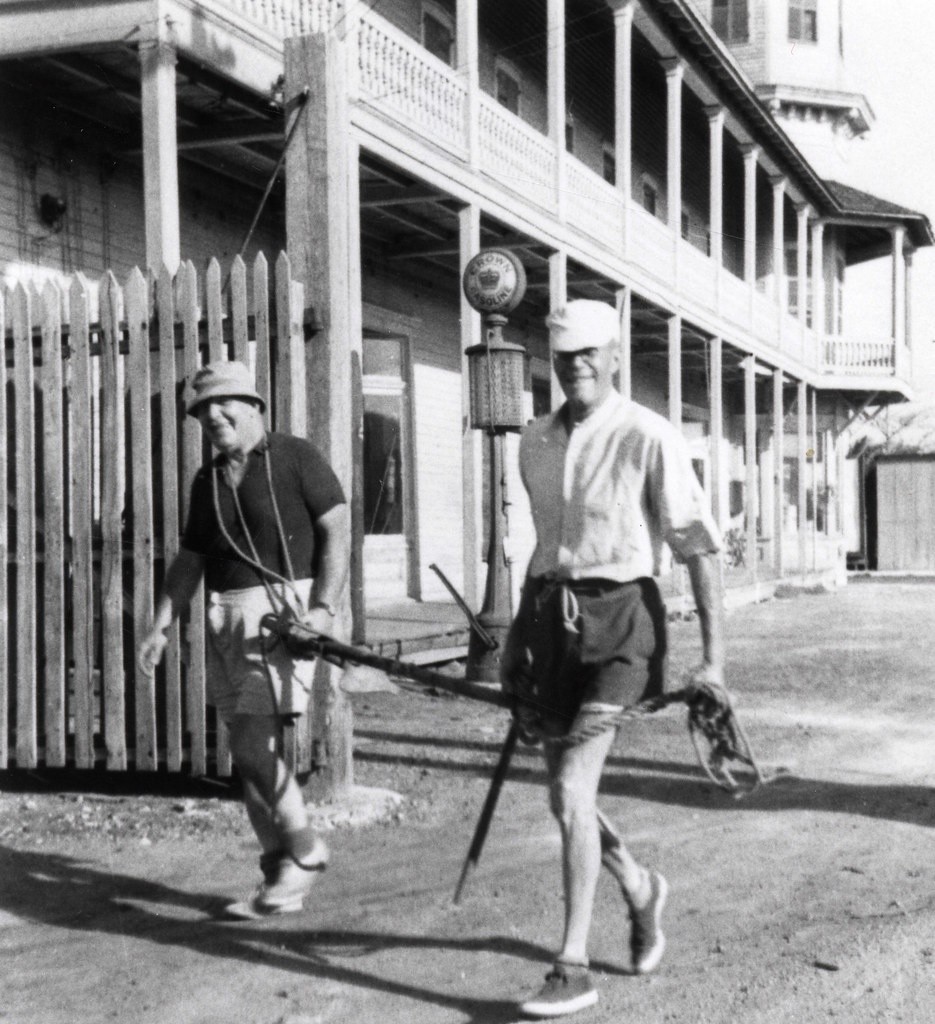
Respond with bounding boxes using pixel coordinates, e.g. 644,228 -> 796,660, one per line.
520,955 -> 601,1017
626,872 -> 670,976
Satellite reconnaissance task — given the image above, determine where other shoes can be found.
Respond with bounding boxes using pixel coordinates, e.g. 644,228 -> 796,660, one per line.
227,884 -> 302,920
263,835 -> 328,906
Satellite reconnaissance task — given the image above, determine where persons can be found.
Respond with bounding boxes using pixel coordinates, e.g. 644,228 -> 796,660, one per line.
497,299 -> 724,1018
135,360 -> 351,923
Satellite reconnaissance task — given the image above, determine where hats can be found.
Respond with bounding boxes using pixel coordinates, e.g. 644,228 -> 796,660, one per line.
185,361 -> 267,415
547,298 -> 621,353
